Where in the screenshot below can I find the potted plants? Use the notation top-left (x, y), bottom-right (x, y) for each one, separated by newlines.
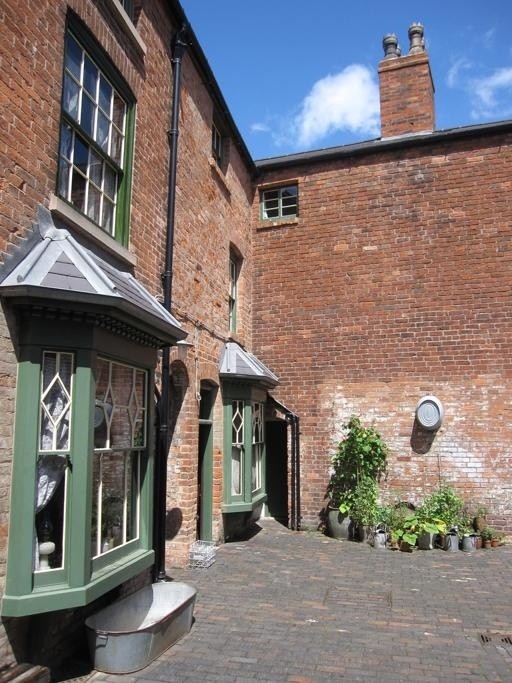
top-left (326, 417), bottom-right (500, 554)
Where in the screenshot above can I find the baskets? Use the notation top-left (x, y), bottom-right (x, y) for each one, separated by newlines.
top-left (189, 540), bottom-right (216, 568)
